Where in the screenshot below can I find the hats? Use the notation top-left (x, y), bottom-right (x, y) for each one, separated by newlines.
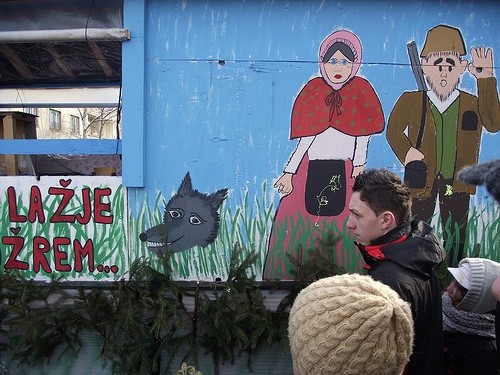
top-left (287, 272), bottom-right (413, 375)
top-left (447, 263), bottom-right (471, 290)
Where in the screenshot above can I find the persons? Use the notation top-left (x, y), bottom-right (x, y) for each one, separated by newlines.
top-left (441, 257), bottom-right (499, 374)
top-left (287, 272), bottom-right (415, 375)
top-left (346, 167), bottom-right (446, 375)
